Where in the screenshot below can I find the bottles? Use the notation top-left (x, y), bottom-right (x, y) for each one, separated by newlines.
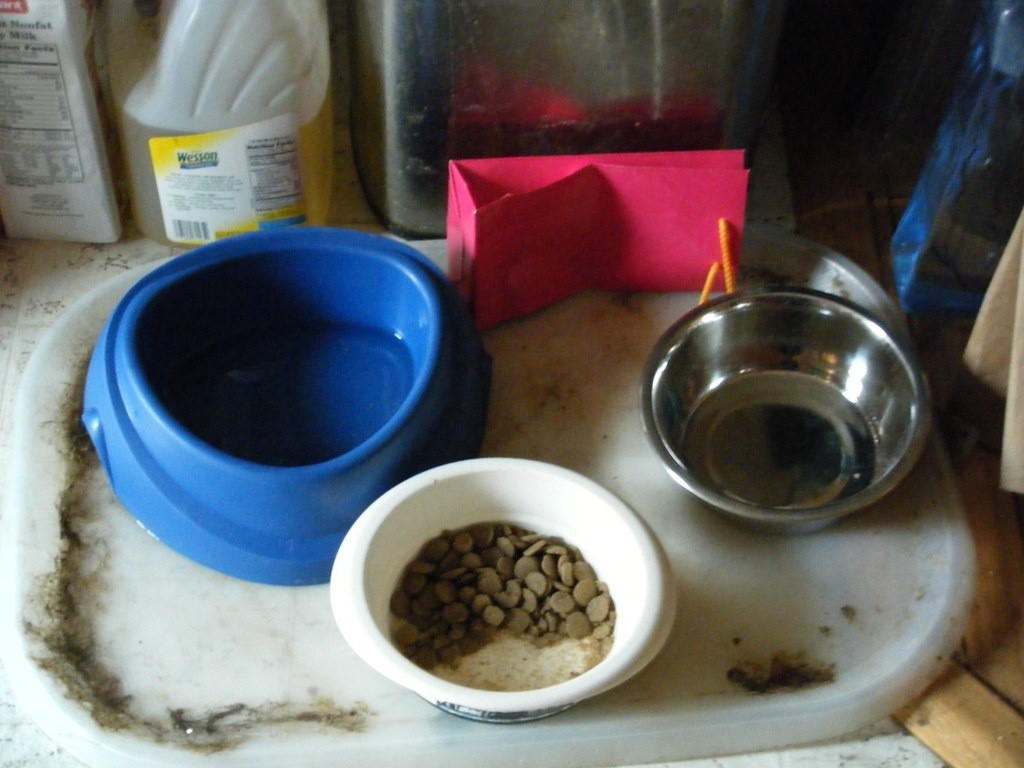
top-left (104, 0), bottom-right (334, 251)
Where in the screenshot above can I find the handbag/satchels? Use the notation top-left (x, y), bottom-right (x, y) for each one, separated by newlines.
top-left (446, 148), bottom-right (749, 335)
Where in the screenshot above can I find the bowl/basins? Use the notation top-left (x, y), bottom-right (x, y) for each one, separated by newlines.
top-left (81, 226), bottom-right (491, 587)
top-left (639, 287), bottom-right (932, 536)
top-left (329, 456), bottom-right (676, 725)
